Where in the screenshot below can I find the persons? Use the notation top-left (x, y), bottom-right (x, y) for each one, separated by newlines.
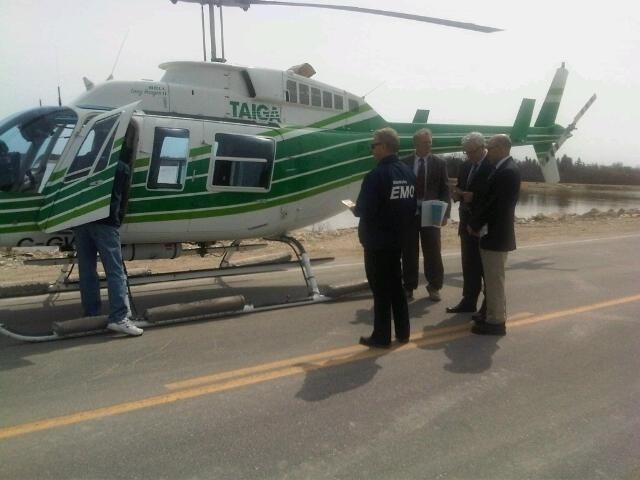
top-left (469, 133), bottom-right (518, 334)
top-left (349, 125), bottom-right (418, 347)
top-left (70, 130), bottom-right (147, 337)
top-left (400, 129), bottom-right (451, 300)
top-left (448, 129), bottom-right (490, 313)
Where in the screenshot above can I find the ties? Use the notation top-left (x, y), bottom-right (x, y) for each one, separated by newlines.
top-left (468, 164), bottom-right (478, 186)
top-left (415, 159), bottom-right (425, 199)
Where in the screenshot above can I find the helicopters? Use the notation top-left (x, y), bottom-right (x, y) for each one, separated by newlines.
top-left (0, 1), bottom-right (599, 342)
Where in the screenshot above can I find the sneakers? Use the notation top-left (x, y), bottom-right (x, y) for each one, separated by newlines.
top-left (107, 318), bottom-right (143, 336)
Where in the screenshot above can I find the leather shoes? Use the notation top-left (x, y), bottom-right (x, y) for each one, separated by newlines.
top-left (359, 285), bottom-right (506, 348)
